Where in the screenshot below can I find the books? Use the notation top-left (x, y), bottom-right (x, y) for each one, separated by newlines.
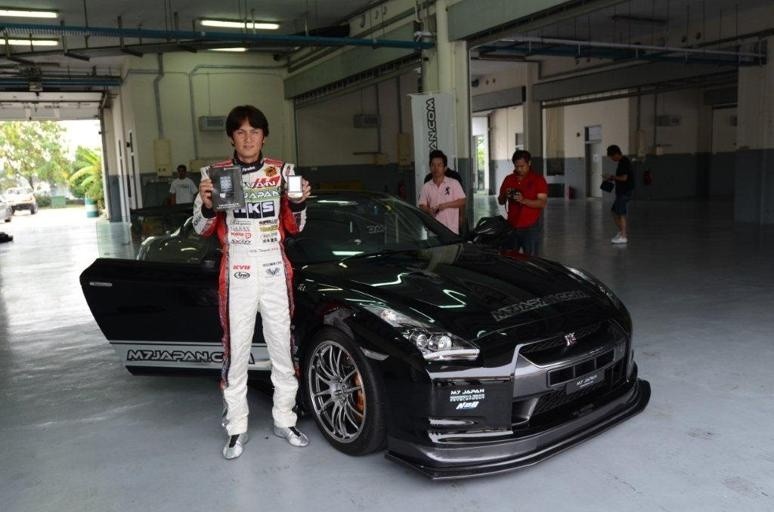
top-left (208, 158), bottom-right (244, 213)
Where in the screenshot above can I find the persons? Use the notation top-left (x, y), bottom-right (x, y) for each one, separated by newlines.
top-left (599, 144), bottom-right (635, 243)
top-left (189, 105), bottom-right (317, 460)
top-left (422, 150), bottom-right (463, 230)
top-left (497, 149), bottom-right (549, 254)
top-left (169, 165), bottom-right (197, 204)
top-left (417, 151), bottom-right (466, 237)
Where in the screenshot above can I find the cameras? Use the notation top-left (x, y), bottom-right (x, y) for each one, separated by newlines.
top-left (507, 188), bottom-right (521, 198)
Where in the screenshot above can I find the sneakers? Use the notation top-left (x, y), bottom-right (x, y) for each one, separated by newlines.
top-left (220, 433), bottom-right (248, 461)
top-left (610, 232), bottom-right (628, 245)
top-left (272, 424), bottom-right (309, 447)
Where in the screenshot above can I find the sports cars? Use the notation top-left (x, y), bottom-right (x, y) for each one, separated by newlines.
top-left (79, 189), bottom-right (651, 480)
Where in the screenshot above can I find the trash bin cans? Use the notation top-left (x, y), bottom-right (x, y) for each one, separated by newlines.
top-left (52, 196), bottom-right (66, 209)
top-left (85, 198), bottom-right (98, 217)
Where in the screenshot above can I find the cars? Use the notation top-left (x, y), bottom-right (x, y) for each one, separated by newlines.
top-left (0, 187), bottom-right (37, 223)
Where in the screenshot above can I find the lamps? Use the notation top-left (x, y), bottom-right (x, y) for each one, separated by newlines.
top-left (0, 37), bottom-right (58, 46)
top-left (198, 16), bottom-right (280, 30)
top-left (0, 6), bottom-right (59, 18)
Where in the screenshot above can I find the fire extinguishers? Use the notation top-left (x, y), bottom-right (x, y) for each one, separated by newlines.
top-left (399, 182), bottom-right (405, 201)
